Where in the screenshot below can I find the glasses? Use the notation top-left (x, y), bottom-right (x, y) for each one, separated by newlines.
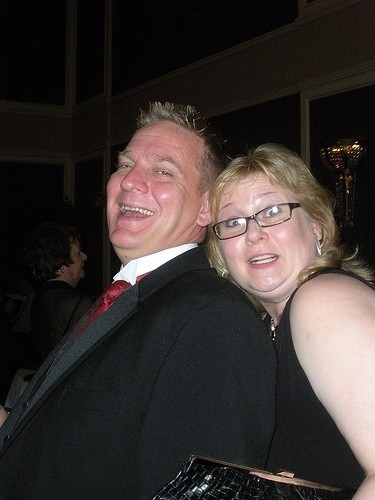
top-left (212, 203), bottom-right (301, 240)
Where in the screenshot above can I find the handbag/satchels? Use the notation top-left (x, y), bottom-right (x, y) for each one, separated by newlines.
top-left (153, 455), bottom-right (357, 500)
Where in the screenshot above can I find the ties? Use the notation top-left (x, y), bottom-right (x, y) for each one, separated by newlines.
top-left (62, 269), bottom-right (153, 351)
